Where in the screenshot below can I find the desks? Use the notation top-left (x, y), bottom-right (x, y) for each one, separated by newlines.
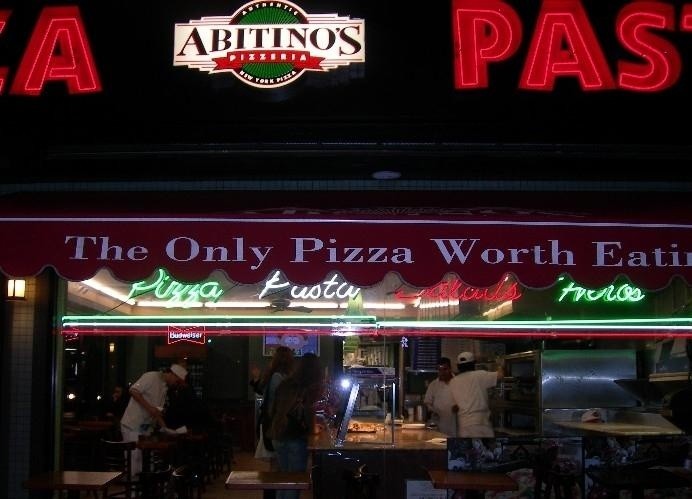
top-left (553, 421), bottom-right (684, 499)
top-left (305, 420), bottom-right (448, 498)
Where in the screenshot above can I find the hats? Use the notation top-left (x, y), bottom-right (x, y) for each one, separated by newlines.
top-left (170, 364), bottom-right (188, 381)
top-left (457, 352), bottom-right (475, 364)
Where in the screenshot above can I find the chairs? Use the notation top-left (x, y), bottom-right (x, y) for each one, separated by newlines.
top-left (99, 420), bottom-right (232, 499)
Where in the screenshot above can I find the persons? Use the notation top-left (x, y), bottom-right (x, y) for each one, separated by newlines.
top-left (424, 351), bottom-right (504, 459)
top-left (120, 364), bottom-right (188, 498)
top-left (262, 346), bottom-right (322, 499)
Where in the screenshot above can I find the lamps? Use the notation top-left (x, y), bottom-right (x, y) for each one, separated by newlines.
top-left (5, 279), bottom-right (26, 299)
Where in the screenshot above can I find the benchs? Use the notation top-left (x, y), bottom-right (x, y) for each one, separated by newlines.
top-left (593, 471), bottom-right (690, 499)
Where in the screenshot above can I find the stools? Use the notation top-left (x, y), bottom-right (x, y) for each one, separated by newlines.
top-left (22, 471), bottom-right (124, 499)
top-left (225, 471), bottom-right (313, 498)
top-left (427, 471), bottom-right (518, 499)
